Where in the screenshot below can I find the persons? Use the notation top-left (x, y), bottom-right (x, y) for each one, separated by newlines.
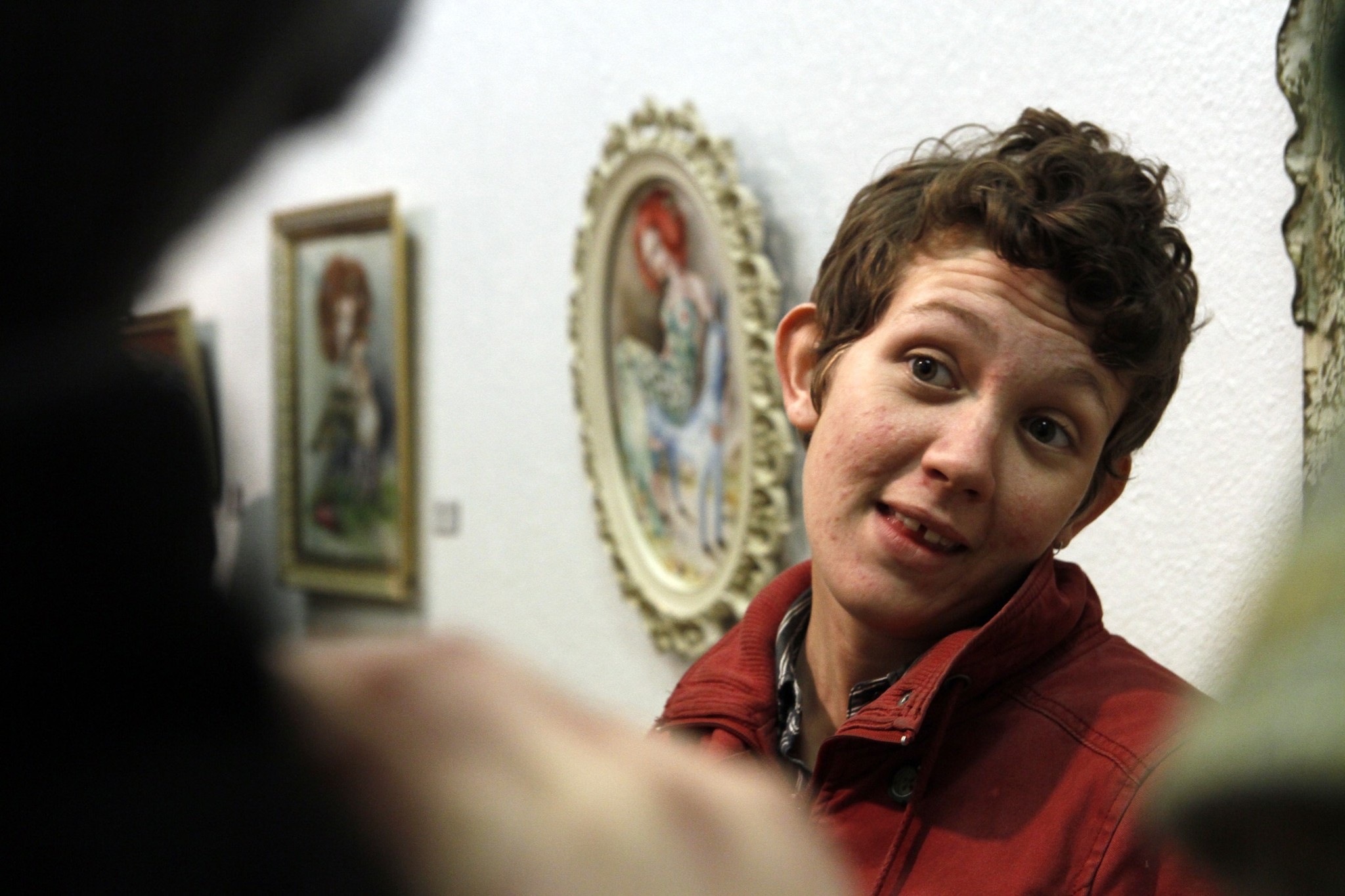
top-left (658, 92), bottom-right (1266, 896)
top-left (0, 1), bottom-right (869, 891)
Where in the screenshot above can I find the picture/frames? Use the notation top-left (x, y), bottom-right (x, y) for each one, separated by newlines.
top-left (119, 307), bottom-right (226, 515)
top-left (564, 90), bottom-right (793, 658)
top-left (266, 190), bottom-right (422, 606)
